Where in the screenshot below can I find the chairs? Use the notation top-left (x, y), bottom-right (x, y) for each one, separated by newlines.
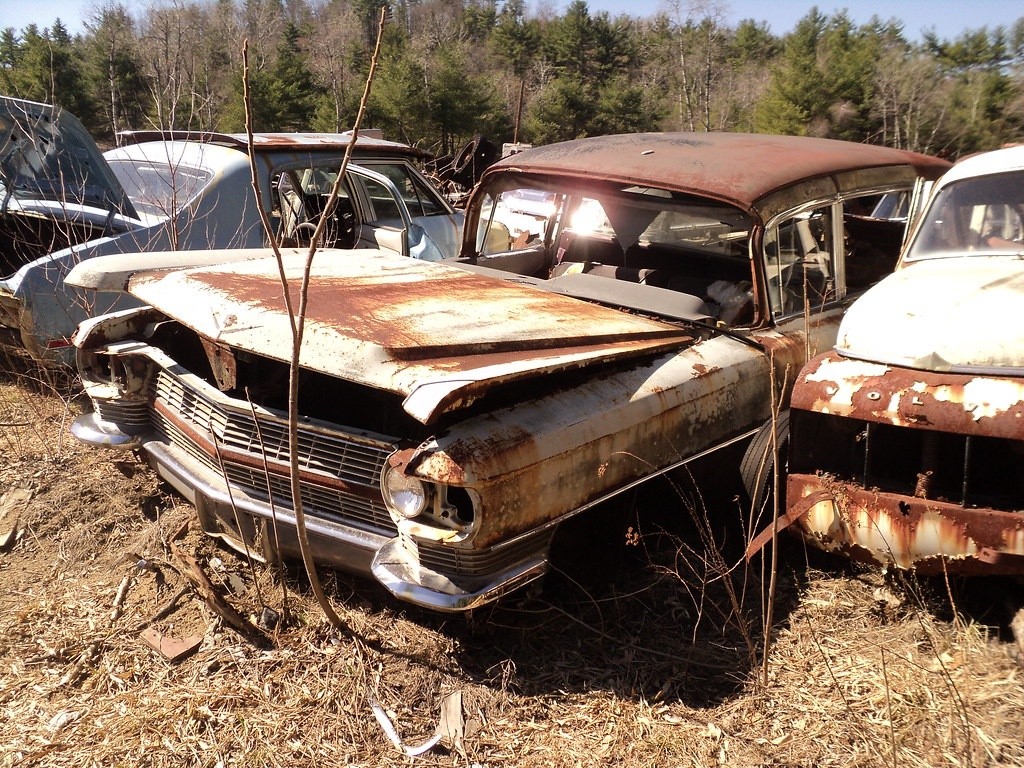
top-left (698, 279), bottom-right (751, 325)
top-left (556, 228), bottom-right (625, 280)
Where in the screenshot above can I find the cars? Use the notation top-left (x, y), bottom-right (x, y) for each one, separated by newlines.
top-left (71, 131), bottom-right (988, 614)
top-left (1, 96), bottom-right (609, 396)
top-left (731, 136), bottom-right (1024, 599)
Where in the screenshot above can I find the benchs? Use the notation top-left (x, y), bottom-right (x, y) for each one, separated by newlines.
top-left (612, 242), bottom-right (750, 289)
